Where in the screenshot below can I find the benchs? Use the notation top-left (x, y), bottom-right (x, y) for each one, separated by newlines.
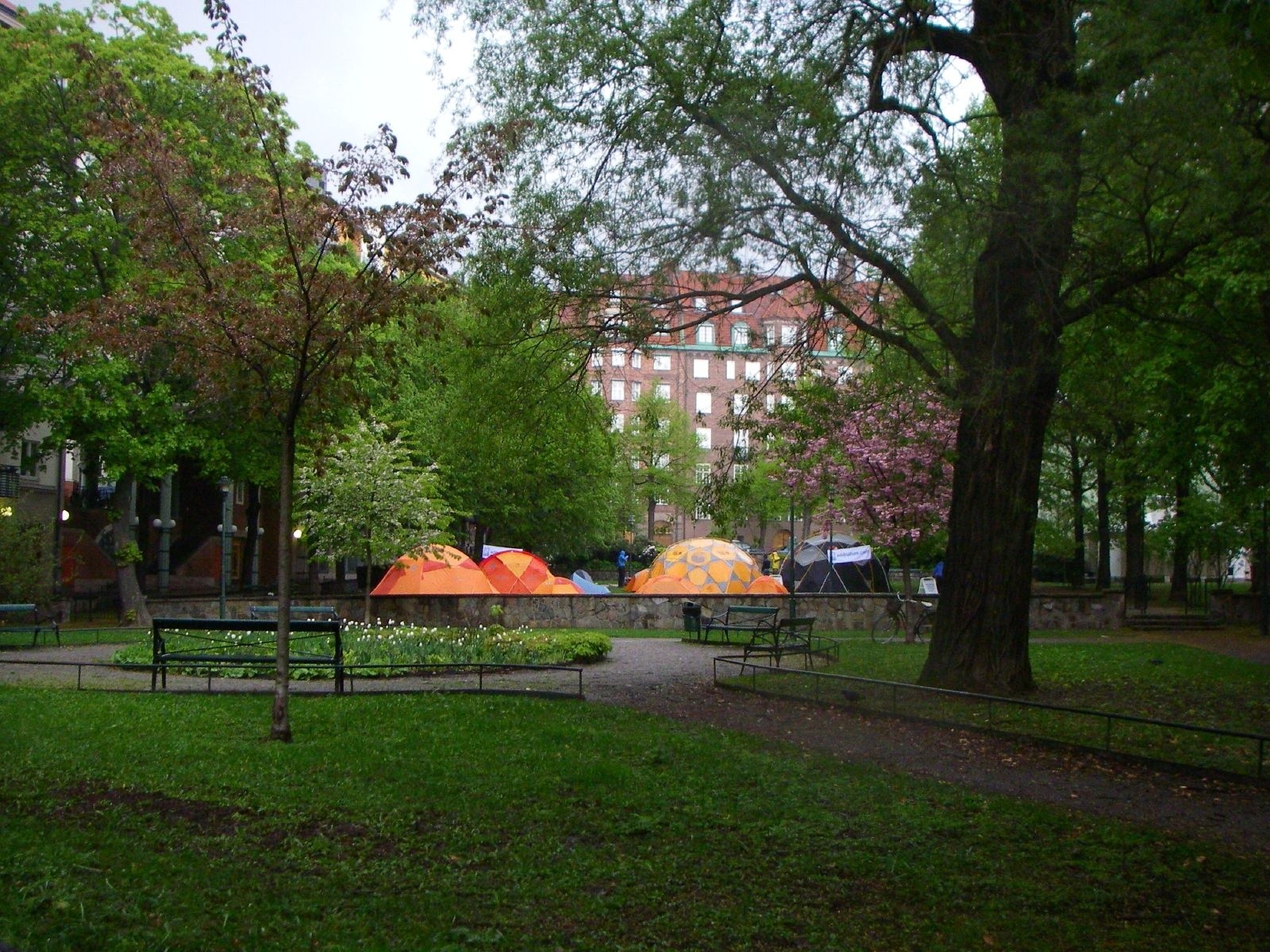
top-left (704, 604), bottom-right (782, 647)
top-left (152, 614), bottom-right (349, 694)
top-left (737, 616), bottom-right (816, 668)
top-left (0, 603), bottom-right (62, 648)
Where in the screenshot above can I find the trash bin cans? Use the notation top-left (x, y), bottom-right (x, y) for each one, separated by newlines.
top-left (682, 601), bottom-right (701, 632)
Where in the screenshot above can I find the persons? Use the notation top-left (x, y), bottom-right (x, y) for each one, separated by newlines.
top-left (750, 550), bottom-right (784, 577)
top-left (932, 558), bottom-right (944, 594)
top-left (617, 549), bottom-right (628, 588)
top-left (882, 553), bottom-right (890, 574)
top-left (644, 542), bottom-right (656, 556)
